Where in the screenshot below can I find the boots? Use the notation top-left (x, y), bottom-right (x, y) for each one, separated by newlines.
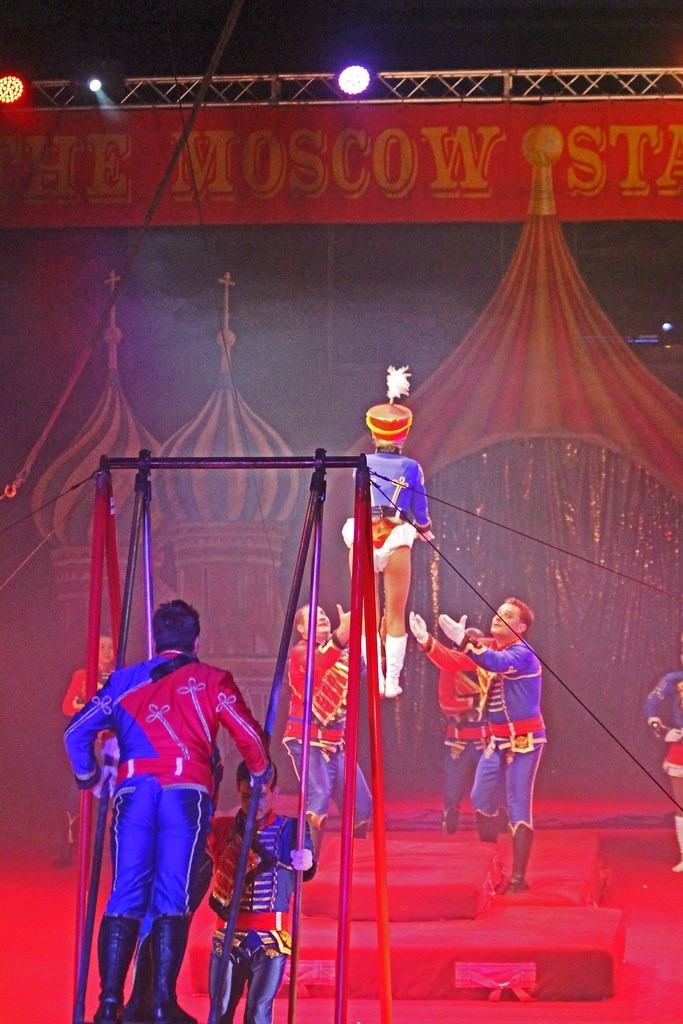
top-left (498, 820), bottom-right (534, 894)
top-left (146, 914), bottom-right (198, 1024)
top-left (306, 812), bottom-right (329, 863)
top-left (51, 811), bottom-right (79, 868)
top-left (672, 816), bottom-right (683, 872)
top-left (475, 810), bottom-right (500, 843)
top-left (353, 820), bottom-right (369, 839)
top-left (122, 932), bottom-right (152, 1024)
top-left (362, 632), bottom-right (385, 694)
top-left (385, 633), bottom-right (409, 698)
top-left (498, 806), bottom-right (509, 833)
top-left (95, 914), bottom-right (139, 1024)
top-left (442, 806), bottom-right (459, 834)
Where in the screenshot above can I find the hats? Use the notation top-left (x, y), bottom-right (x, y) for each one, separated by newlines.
top-left (366, 365), bottom-right (413, 446)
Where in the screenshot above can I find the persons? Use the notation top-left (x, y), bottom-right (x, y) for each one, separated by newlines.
top-left (61, 596), bottom-right (548, 1024)
top-left (642, 652), bottom-right (683, 872)
top-left (340, 404), bottom-right (436, 699)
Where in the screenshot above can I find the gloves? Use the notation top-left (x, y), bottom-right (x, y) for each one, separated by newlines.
top-left (409, 611), bottom-right (430, 645)
top-left (291, 849), bottom-right (313, 870)
top-left (416, 530), bottom-right (435, 543)
top-left (249, 760), bottom-right (274, 794)
top-left (438, 614), bottom-right (468, 646)
top-left (92, 766), bottom-right (117, 799)
